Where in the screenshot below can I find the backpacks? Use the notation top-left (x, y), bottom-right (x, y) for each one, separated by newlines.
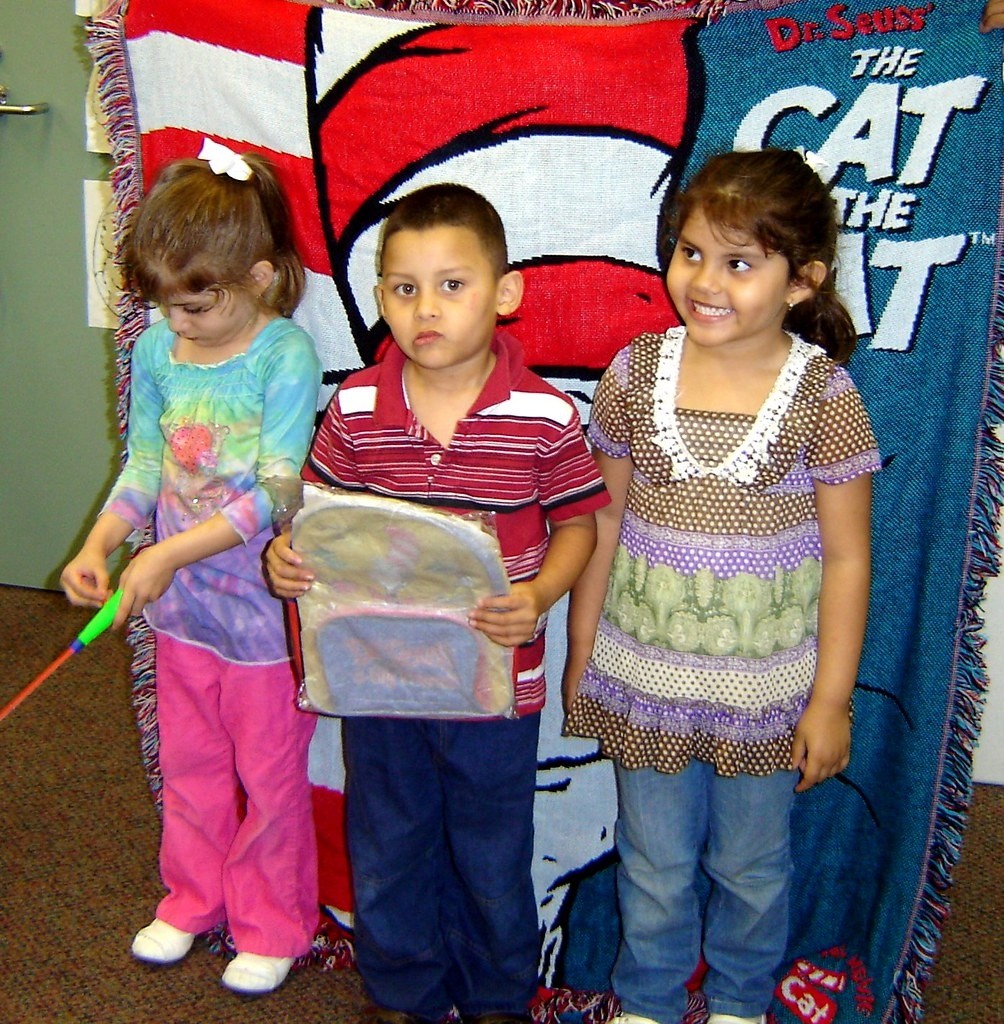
top-left (291, 501), bottom-right (513, 716)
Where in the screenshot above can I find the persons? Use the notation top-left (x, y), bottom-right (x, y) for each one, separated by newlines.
top-left (565, 149), bottom-right (882, 1024)
top-left (61, 137), bottom-right (324, 992)
top-left (268, 184), bottom-right (613, 1024)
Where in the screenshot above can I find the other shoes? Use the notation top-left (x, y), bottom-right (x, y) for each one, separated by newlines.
top-left (132, 917), bottom-right (197, 963)
top-left (223, 951), bottom-right (295, 993)
